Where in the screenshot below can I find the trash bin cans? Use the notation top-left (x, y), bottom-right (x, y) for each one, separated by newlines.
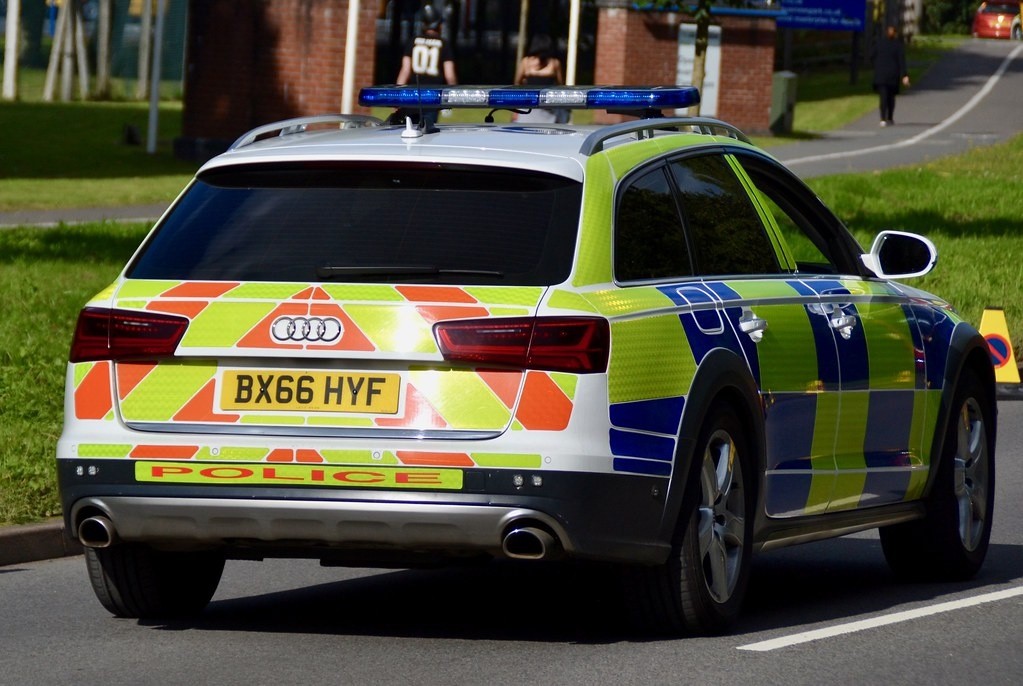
top-left (769, 70), bottom-right (798, 138)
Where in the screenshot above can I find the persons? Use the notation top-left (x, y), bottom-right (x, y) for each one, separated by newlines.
top-left (397, 15), bottom-right (456, 122)
top-left (511, 33), bottom-right (563, 124)
top-left (870, 25), bottom-right (910, 128)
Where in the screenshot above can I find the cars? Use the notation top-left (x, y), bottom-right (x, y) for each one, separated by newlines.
top-left (972, 0), bottom-right (1023, 40)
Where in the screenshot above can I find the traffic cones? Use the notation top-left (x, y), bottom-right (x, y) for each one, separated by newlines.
top-left (978, 305), bottom-right (1023, 401)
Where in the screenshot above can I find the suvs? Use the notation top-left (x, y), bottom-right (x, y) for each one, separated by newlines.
top-left (55, 84), bottom-right (997, 632)
